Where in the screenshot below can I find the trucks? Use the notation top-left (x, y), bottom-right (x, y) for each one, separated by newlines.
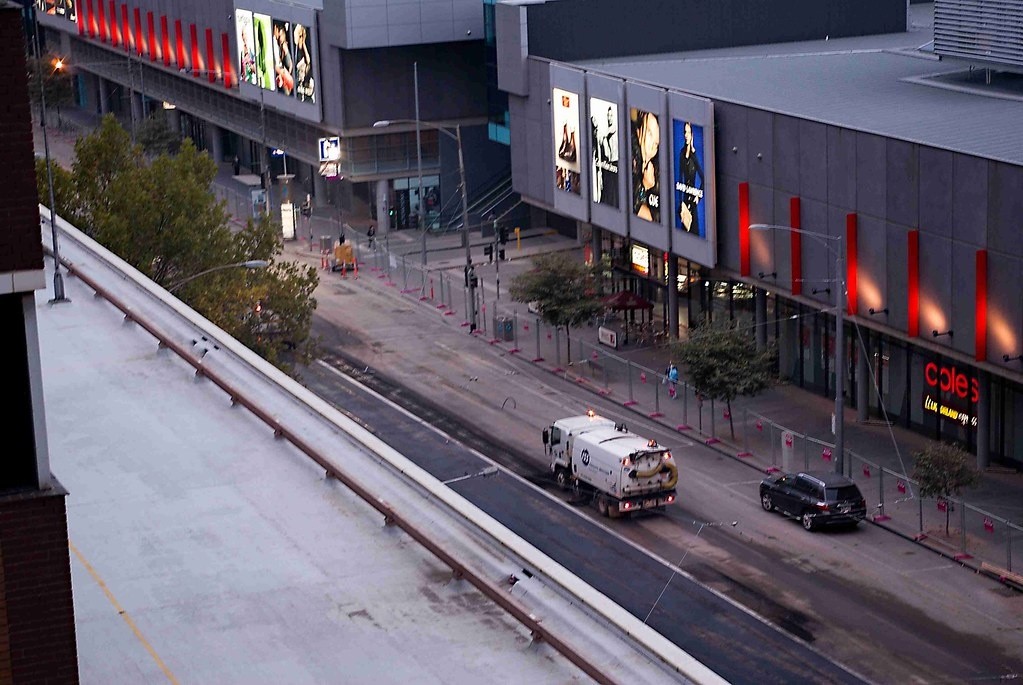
top-left (543, 408), bottom-right (679, 520)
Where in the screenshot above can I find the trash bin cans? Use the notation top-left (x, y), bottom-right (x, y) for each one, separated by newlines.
top-left (497, 315), bottom-right (513, 340)
top-left (425, 211), bottom-right (439, 231)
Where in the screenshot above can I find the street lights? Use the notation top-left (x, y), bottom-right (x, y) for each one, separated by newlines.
top-left (372, 118), bottom-right (474, 270)
top-left (179, 65), bottom-right (273, 219)
top-left (52, 53), bottom-right (148, 124)
top-left (748, 218), bottom-right (849, 475)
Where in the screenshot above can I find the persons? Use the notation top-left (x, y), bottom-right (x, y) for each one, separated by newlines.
top-left (296, 64), bottom-right (315, 102)
top-left (676, 122), bottom-right (704, 236)
top-left (664, 359), bottom-right (680, 400)
top-left (601, 107), bottom-right (619, 208)
top-left (293, 24), bottom-right (311, 74)
top-left (273, 22), bottom-right (284, 93)
top-left (238, 30), bottom-right (255, 85)
top-left (627, 113), bottom-right (660, 223)
top-left (590, 116), bottom-right (607, 203)
top-left (279, 26), bottom-right (293, 95)
top-left (367, 225), bottom-right (377, 252)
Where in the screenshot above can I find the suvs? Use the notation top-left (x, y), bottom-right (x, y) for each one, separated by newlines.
top-left (757, 470), bottom-right (868, 531)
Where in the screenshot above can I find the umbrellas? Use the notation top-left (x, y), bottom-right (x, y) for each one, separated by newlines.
top-left (597, 289), bottom-right (654, 346)
